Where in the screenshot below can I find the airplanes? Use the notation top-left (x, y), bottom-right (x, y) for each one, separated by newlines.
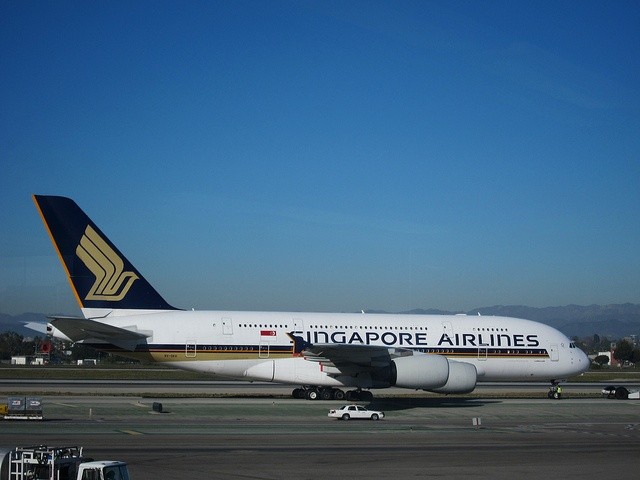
top-left (31, 194), bottom-right (590, 400)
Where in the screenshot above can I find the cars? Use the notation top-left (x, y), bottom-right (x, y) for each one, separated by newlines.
top-left (328, 404), bottom-right (384, 421)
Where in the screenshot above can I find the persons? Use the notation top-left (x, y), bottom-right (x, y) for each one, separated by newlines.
top-left (556, 385), bottom-right (562, 400)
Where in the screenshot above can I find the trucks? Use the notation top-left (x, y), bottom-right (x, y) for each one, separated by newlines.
top-left (0, 444), bottom-right (130, 480)
top-left (0, 396), bottom-right (43, 421)
